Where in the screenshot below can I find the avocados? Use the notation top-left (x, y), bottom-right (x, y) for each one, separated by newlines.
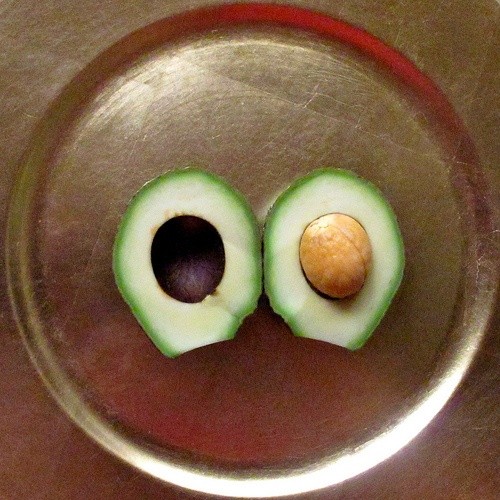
top-left (113, 164), bottom-right (404, 360)
